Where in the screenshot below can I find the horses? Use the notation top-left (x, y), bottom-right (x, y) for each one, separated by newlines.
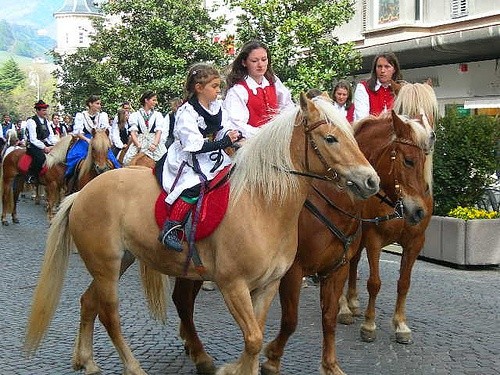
top-left (22, 92), bottom-right (381, 375)
top-left (0, 124), bottom-right (158, 224)
top-left (335, 78), bottom-right (440, 345)
top-left (171, 109), bottom-right (436, 375)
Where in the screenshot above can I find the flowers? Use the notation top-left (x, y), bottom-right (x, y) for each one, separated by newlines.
top-left (446, 205), bottom-right (500, 221)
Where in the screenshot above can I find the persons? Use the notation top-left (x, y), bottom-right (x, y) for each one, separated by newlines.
top-left (64, 95), bottom-right (123, 188)
top-left (61, 114), bottom-right (74, 134)
top-left (331, 81), bottom-right (355, 125)
top-left (50, 113), bottom-right (68, 143)
top-left (112, 109), bottom-right (131, 159)
top-left (353, 53), bottom-right (403, 123)
top-left (109, 117), bottom-right (114, 126)
top-left (158, 62), bottom-right (246, 253)
top-left (0, 115), bottom-right (31, 145)
top-left (122, 91), bottom-right (167, 167)
top-left (112, 102), bottom-right (131, 125)
top-left (165, 96), bottom-right (184, 147)
top-left (223, 41), bottom-right (296, 150)
top-left (24, 99), bottom-right (55, 187)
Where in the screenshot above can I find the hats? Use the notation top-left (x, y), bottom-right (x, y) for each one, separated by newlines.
top-left (34, 100), bottom-right (49, 109)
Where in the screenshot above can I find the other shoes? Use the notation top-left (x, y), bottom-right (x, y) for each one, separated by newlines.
top-left (64, 176), bottom-right (74, 195)
top-left (158, 218), bottom-right (185, 252)
top-left (28, 172), bottom-right (39, 184)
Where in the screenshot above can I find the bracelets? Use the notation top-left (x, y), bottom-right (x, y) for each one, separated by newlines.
top-left (152, 143), bottom-right (157, 147)
